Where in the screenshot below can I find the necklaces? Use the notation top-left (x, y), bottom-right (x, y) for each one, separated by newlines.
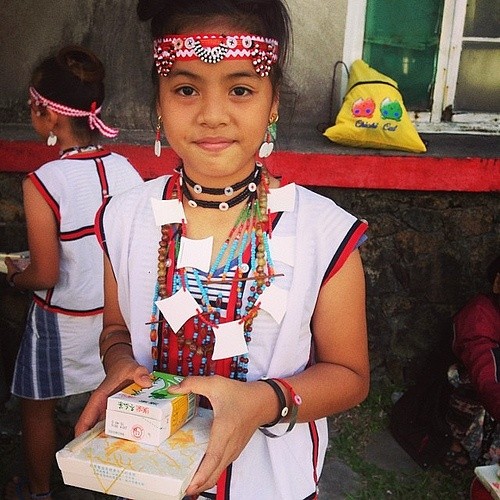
top-left (149, 159), bottom-right (275, 411)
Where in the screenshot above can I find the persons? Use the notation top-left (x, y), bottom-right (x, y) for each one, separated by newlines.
top-left (0, 46), bottom-right (144, 500)
top-left (428, 257), bottom-right (500, 500)
top-left (74, 0), bottom-right (369, 499)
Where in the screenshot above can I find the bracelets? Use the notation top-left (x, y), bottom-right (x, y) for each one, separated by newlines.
top-left (99, 327), bottom-right (132, 364)
top-left (257, 378), bottom-right (301, 438)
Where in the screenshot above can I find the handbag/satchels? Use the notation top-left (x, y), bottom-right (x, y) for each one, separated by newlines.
top-left (323, 57), bottom-right (427, 153)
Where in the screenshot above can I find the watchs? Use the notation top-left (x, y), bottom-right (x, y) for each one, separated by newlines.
top-left (9, 271), bottom-right (22, 287)
